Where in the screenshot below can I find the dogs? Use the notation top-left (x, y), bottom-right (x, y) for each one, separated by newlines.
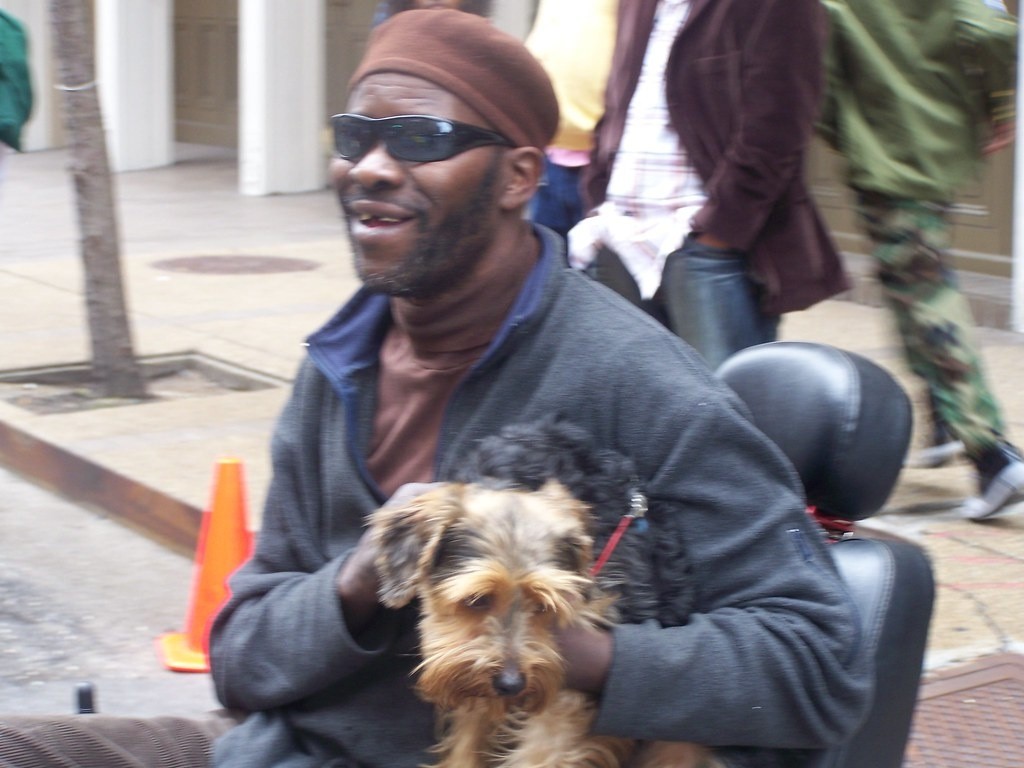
top-left (361, 415), bottom-right (733, 768)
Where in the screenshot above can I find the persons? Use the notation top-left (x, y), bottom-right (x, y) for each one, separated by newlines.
top-left (201, 11), bottom-right (876, 768)
top-left (523, 2), bottom-right (1024, 525)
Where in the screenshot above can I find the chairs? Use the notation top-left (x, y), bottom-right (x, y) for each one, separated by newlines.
top-left (713, 343), bottom-right (938, 768)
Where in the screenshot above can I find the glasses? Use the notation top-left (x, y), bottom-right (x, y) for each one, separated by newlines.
top-left (330, 109), bottom-right (517, 166)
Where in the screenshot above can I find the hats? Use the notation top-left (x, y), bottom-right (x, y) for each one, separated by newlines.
top-left (347, 8), bottom-right (560, 151)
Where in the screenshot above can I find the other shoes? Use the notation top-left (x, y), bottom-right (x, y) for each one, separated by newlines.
top-left (955, 462), bottom-right (1024, 519)
top-left (901, 431), bottom-right (971, 469)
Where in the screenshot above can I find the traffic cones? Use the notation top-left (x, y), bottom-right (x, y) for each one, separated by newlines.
top-left (160, 457), bottom-right (256, 672)
